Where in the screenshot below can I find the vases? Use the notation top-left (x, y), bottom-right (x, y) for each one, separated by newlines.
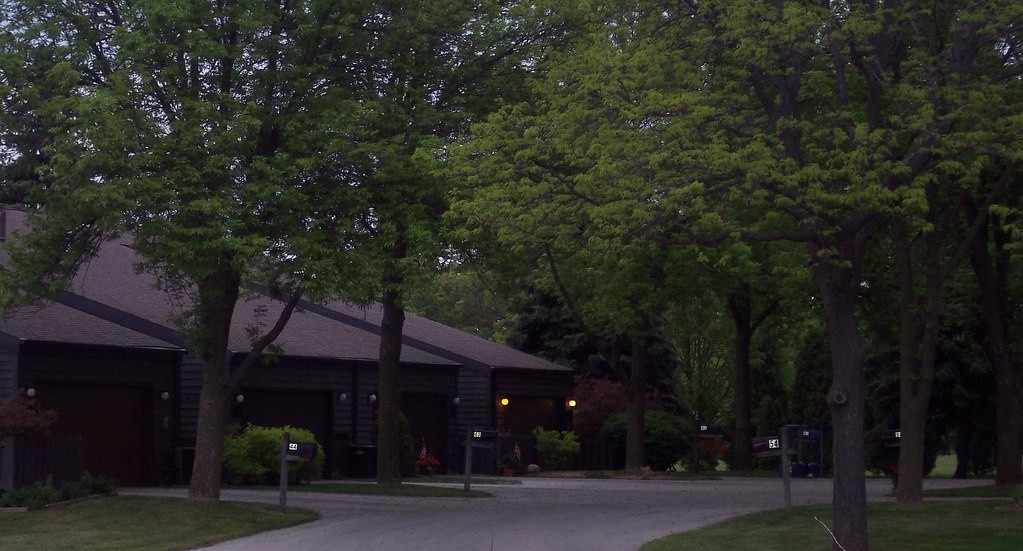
top-left (502, 465), bottom-right (517, 476)
top-left (420, 465), bottom-right (435, 475)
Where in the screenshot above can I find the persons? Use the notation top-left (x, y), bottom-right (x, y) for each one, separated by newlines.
top-left (885, 463), bottom-right (898, 488)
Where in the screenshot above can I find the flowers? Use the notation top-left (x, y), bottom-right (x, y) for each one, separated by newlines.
top-left (414, 456), bottom-right (440, 467)
top-left (495, 450), bottom-right (513, 465)
